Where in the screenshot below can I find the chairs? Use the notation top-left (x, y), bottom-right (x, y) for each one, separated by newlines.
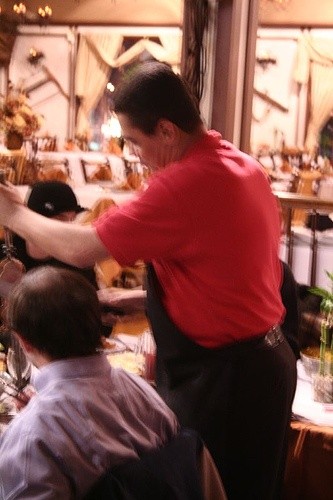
top-left (72, 426), bottom-right (206, 500)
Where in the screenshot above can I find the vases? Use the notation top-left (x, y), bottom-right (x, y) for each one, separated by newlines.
top-left (3, 131), bottom-right (22, 149)
top-left (300, 347), bottom-right (333, 402)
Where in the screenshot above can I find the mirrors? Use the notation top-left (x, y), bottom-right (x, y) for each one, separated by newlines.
top-left (0, 0), bottom-right (333, 208)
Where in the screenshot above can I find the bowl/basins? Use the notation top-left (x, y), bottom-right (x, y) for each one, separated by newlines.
top-left (299, 350), bottom-right (333, 376)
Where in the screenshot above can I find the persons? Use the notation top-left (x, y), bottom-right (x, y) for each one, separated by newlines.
top-left (0, 181), bottom-right (100, 291)
top-left (145, 257), bottom-right (301, 401)
top-left (0, 63), bottom-right (297, 500)
top-left (1, 264), bottom-right (181, 500)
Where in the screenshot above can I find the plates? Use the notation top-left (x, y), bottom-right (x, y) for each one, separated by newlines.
top-left (105, 352), bottom-right (146, 375)
top-left (96, 338), bottom-right (125, 353)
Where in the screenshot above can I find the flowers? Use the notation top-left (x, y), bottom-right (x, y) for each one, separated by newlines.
top-left (1, 90), bottom-right (41, 138)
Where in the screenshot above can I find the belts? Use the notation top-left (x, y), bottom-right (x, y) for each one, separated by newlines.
top-left (202, 322), bottom-right (287, 358)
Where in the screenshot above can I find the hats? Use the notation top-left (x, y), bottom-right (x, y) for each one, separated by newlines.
top-left (28, 180), bottom-right (87, 219)
